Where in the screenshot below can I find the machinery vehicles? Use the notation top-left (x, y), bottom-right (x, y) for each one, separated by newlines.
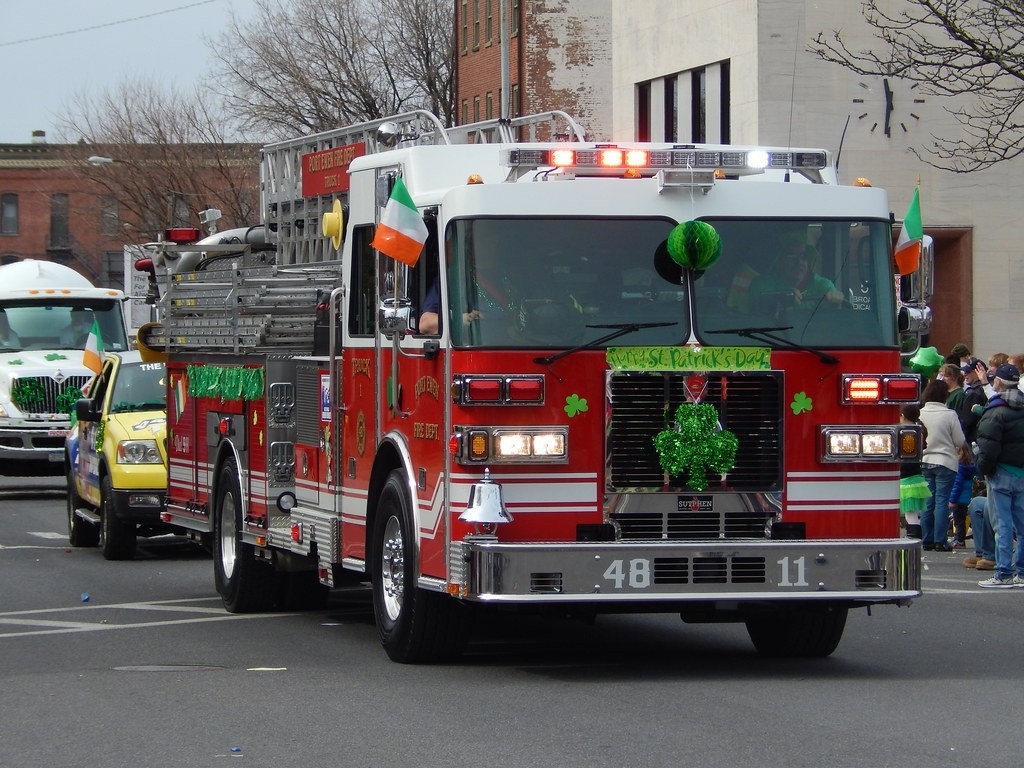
top-left (0, 258), bottom-right (129, 478)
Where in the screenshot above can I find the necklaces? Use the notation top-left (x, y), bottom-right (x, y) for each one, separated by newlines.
top-left (471, 267), bottom-right (517, 314)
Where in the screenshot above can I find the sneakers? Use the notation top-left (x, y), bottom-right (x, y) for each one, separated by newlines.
top-left (978, 577), bottom-right (1013, 588)
top-left (951, 540), bottom-right (966, 549)
top-left (924, 542), bottom-right (951, 552)
top-left (963, 557), bottom-right (995, 570)
top-left (1013, 575), bottom-right (1024, 588)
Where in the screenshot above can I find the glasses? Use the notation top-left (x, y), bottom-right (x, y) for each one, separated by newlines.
top-left (788, 252), bottom-right (806, 260)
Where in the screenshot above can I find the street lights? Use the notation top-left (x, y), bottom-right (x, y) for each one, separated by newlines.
top-left (89, 155), bottom-right (166, 245)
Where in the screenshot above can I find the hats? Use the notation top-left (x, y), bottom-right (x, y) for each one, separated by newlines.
top-left (951, 344), bottom-right (971, 357)
top-left (957, 359), bottom-right (988, 372)
top-left (994, 364), bottom-right (1020, 382)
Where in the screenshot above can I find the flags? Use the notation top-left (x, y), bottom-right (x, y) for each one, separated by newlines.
top-left (894, 187), bottom-right (923, 277)
top-left (83, 318), bottom-right (106, 376)
top-left (368, 172), bottom-right (429, 268)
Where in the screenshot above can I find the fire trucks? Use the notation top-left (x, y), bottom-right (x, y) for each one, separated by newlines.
top-left (131, 109), bottom-right (948, 659)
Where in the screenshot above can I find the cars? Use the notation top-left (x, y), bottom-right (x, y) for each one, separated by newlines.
top-left (65, 348), bottom-right (171, 560)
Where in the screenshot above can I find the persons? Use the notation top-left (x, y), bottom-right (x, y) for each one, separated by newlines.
top-left (0, 307), bottom-right (21, 350)
top-left (899, 341), bottom-right (1024, 589)
top-left (60, 307), bottom-right (92, 345)
top-left (750, 237), bottom-right (837, 318)
top-left (418, 220), bottom-right (583, 335)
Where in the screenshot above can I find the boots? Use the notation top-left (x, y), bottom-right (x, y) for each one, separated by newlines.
top-left (906, 524), bottom-right (923, 541)
